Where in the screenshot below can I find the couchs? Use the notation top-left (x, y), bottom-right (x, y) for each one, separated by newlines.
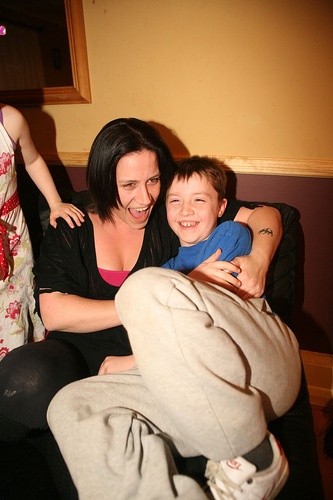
top-left (19, 168), bottom-right (333, 500)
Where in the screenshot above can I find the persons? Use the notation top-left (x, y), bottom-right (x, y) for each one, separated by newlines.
top-left (45, 155), bottom-right (303, 500)
top-left (0, 116), bottom-right (282, 500)
top-left (0, 104), bottom-right (86, 363)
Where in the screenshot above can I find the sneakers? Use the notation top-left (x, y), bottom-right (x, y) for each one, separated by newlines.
top-left (204, 428), bottom-right (290, 500)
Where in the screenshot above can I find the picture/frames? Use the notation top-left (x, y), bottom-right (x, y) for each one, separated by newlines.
top-left (0, 2), bottom-right (93, 106)
